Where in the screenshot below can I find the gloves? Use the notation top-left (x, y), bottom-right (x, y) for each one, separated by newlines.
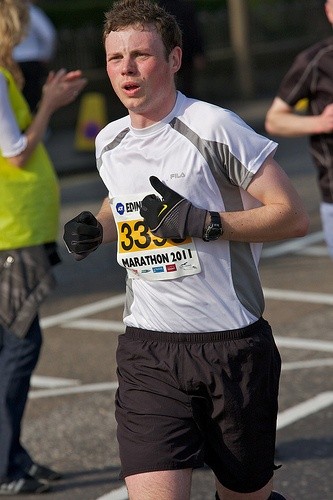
top-left (62, 211), bottom-right (104, 260)
top-left (138, 176), bottom-right (206, 241)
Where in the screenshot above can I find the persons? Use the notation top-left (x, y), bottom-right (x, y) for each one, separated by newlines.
top-left (0, 0), bottom-right (88, 496)
top-left (264, 0), bottom-right (333, 260)
top-left (62, 0), bottom-right (309, 500)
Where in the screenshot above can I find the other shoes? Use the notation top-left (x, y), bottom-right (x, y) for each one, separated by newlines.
top-left (0, 474), bottom-right (50, 496)
top-left (28, 462), bottom-right (62, 482)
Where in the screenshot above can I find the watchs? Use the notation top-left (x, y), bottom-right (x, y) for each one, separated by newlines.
top-left (203, 210), bottom-right (224, 243)
